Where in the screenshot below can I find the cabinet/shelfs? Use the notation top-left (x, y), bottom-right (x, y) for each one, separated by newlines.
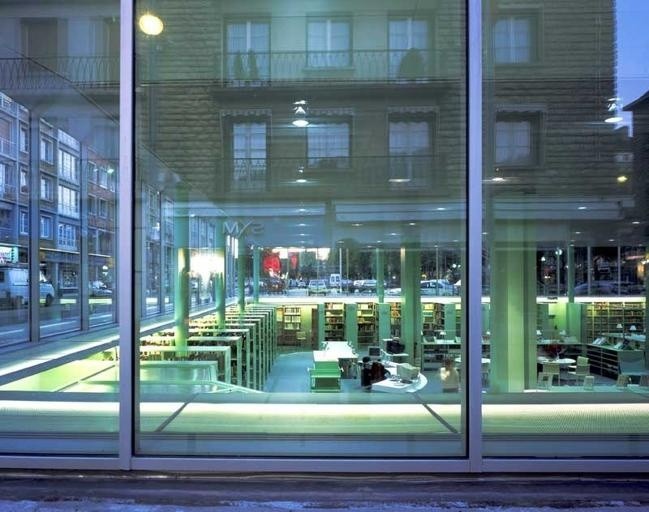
top-left (87, 303), bottom-right (277, 391)
top-left (537, 301), bottom-right (649, 383)
top-left (421, 339), bottom-right (491, 371)
top-left (275, 302), bottom-right (461, 350)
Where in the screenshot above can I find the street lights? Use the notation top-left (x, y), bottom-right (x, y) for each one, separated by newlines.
top-left (540, 257), bottom-right (546, 276)
top-left (553, 247), bottom-right (563, 297)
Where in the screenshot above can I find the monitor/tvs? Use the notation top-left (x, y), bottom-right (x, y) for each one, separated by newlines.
top-left (397, 363), bottom-right (419, 383)
top-left (367, 346), bottom-right (383, 360)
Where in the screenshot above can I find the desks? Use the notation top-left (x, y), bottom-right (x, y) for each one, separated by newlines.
top-left (380, 348), bottom-right (409, 363)
top-left (322, 340), bottom-right (357, 377)
top-left (307, 350), bottom-right (342, 393)
top-left (454, 357), bottom-right (491, 387)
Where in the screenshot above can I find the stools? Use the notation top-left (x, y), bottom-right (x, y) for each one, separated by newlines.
top-left (481, 370), bottom-right (489, 386)
top-left (583, 376), bottom-right (595, 390)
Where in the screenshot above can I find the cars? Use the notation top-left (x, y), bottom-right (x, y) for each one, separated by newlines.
top-left (190, 270), bottom-right (457, 298)
top-left (563, 253), bottom-right (646, 296)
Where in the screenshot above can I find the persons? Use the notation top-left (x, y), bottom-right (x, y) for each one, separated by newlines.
top-left (264, 279), bottom-right (289, 296)
top-left (363, 357), bottom-right (391, 393)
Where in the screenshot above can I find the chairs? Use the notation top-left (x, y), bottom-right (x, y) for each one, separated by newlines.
top-left (538, 363), bottom-right (560, 390)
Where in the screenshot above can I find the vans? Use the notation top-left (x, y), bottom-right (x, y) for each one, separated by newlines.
top-left (0, 261), bottom-right (55, 307)
